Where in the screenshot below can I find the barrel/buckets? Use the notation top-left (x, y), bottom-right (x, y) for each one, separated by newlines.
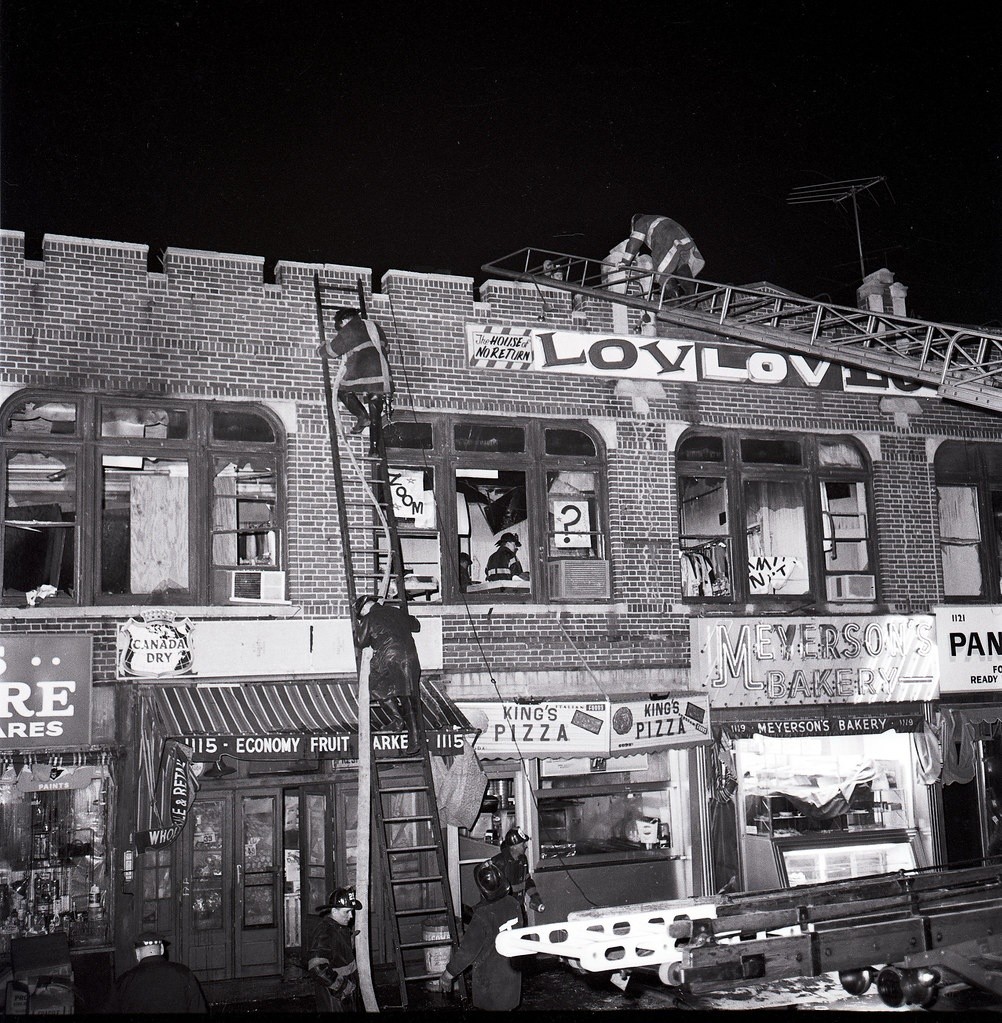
top-left (423, 915), bottom-right (460, 992)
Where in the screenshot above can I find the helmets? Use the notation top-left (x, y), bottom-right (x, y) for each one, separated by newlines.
top-left (473, 860), bottom-right (509, 901)
top-left (504, 827), bottom-right (530, 846)
top-left (314, 888), bottom-right (362, 910)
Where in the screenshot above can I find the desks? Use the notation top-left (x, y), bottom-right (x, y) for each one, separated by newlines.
top-left (465, 580), bottom-right (530, 594)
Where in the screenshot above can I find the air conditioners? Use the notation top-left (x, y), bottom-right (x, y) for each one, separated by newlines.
top-left (825, 574), bottom-right (877, 602)
top-left (545, 559), bottom-right (612, 602)
top-left (229, 569), bottom-right (288, 603)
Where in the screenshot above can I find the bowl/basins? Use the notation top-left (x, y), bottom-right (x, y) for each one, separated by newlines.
top-left (779, 812), bottom-right (792, 816)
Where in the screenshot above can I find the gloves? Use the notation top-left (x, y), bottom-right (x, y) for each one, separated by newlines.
top-left (530, 894), bottom-right (542, 905)
top-left (346, 987), bottom-right (359, 1002)
top-left (438, 975), bottom-right (452, 992)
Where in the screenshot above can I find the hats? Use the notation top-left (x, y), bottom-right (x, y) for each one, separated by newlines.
top-left (134, 932), bottom-right (163, 948)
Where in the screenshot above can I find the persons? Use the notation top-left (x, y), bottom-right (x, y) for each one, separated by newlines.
top-left (354, 595), bottom-right (421, 755)
top-left (438, 827), bottom-right (544, 1012)
top-left (459, 552), bottom-right (482, 592)
top-left (99, 931), bottom-right (208, 1013)
top-left (616, 213), bottom-right (706, 311)
top-left (543, 260), bottom-right (563, 281)
top-left (307, 887), bottom-right (362, 1012)
top-left (485, 532), bottom-right (530, 582)
top-left (316, 307), bottom-right (394, 458)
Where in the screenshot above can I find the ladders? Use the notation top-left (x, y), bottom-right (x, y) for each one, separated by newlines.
top-left (311, 269), bottom-right (467, 1008)
